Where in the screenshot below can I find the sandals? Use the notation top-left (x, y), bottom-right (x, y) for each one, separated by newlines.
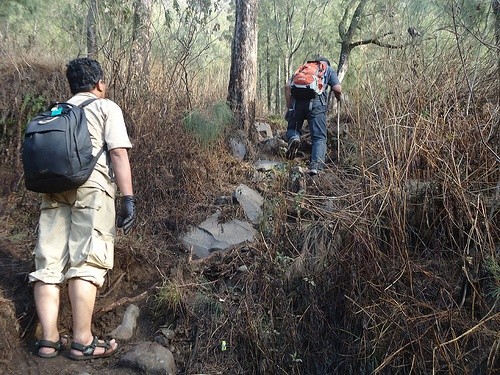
top-left (68, 335), bottom-right (119, 359)
top-left (34, 334), bottom-right (71, 358)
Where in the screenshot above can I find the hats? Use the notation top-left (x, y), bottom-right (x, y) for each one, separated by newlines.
top-left (315, 57), bottom-right (330, 66)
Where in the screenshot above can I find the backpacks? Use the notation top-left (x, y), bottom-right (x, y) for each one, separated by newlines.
top-left (22, 98), bottom-right (109, 193)
top-left (289, 60), bottom-right (329, 99)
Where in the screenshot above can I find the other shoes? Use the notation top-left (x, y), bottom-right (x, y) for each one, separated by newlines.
top-left (285, 138), bottom-right (300, 160)
top-left (309, 168), bottom-right (325, 176)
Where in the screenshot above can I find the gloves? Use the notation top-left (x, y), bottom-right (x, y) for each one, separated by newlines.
top-left (285, 106), bottom-right (294, 122)
top-left (117, 195), bottom-right (136, 235)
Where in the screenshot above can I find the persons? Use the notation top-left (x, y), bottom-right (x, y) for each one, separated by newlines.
top-left (29, 60), bottom-right (136, 360)
top-left (283, 57), bottom-right (343, 174)
